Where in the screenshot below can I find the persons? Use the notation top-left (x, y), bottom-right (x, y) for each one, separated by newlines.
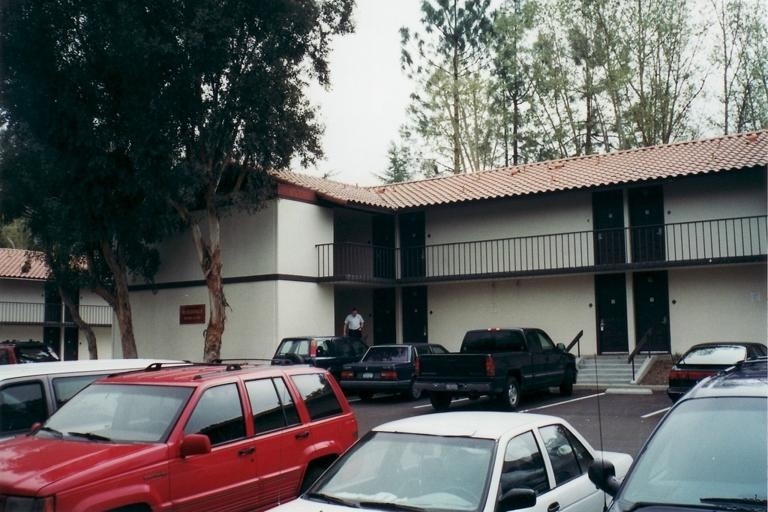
top-left (343, 308), bottom-right (365, 341)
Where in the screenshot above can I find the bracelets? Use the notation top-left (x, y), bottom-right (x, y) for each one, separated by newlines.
top-left (360, 327), bottom-right (362, 329)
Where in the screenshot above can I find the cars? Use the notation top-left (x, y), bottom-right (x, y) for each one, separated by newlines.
top-left (603, 356), bottom-right (767, 512)
top-left (265, 408), bottom-right (632, 512)
top-left (416, 327), bottom-right (577, 410)
top-left (669, 340), bottom-right (767, 403)
top-left (270, 336), bottom-right (449, 400)
top-left (0, 340), bottom-right (60, 363)
